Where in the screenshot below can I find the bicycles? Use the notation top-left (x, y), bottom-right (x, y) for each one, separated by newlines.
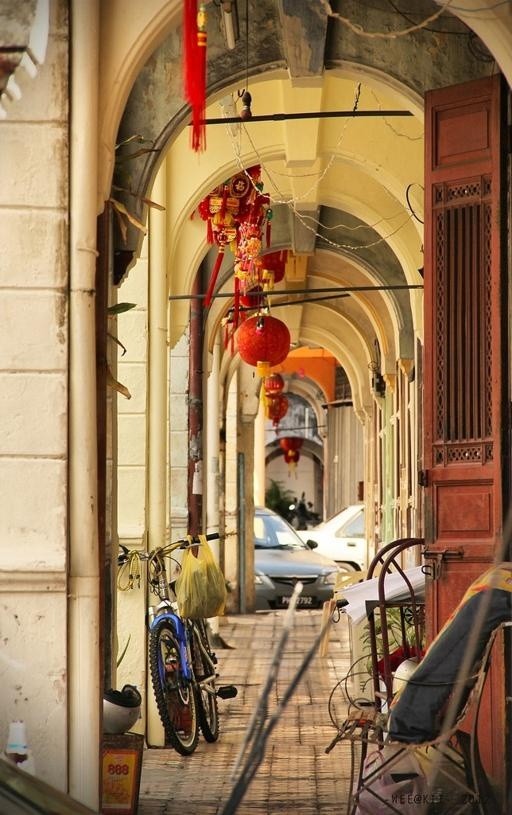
top-left (118, 532), bottom-right (238, 758)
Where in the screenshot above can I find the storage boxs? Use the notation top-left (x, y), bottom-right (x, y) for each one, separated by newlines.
top-left (101, 733), bottom-right (145, 814)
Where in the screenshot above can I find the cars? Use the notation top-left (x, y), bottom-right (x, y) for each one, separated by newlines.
top-left (251, 505), bottom-right (343, 608)
top-left (268, 504), bottom-right (367, 590)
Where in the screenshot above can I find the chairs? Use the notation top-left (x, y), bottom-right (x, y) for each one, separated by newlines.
top-left (324, 559), bottom-right (512, 814)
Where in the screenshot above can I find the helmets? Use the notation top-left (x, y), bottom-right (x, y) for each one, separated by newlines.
top-left (101, 686), bottom-right (142, 735)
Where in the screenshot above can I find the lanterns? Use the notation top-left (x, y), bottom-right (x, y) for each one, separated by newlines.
top-left (280, 436), bottom-right (305, 457)
top-left (282, 451), bottom-right (301, 479)
top-left (195, 161), bottom-right (290, 428)
